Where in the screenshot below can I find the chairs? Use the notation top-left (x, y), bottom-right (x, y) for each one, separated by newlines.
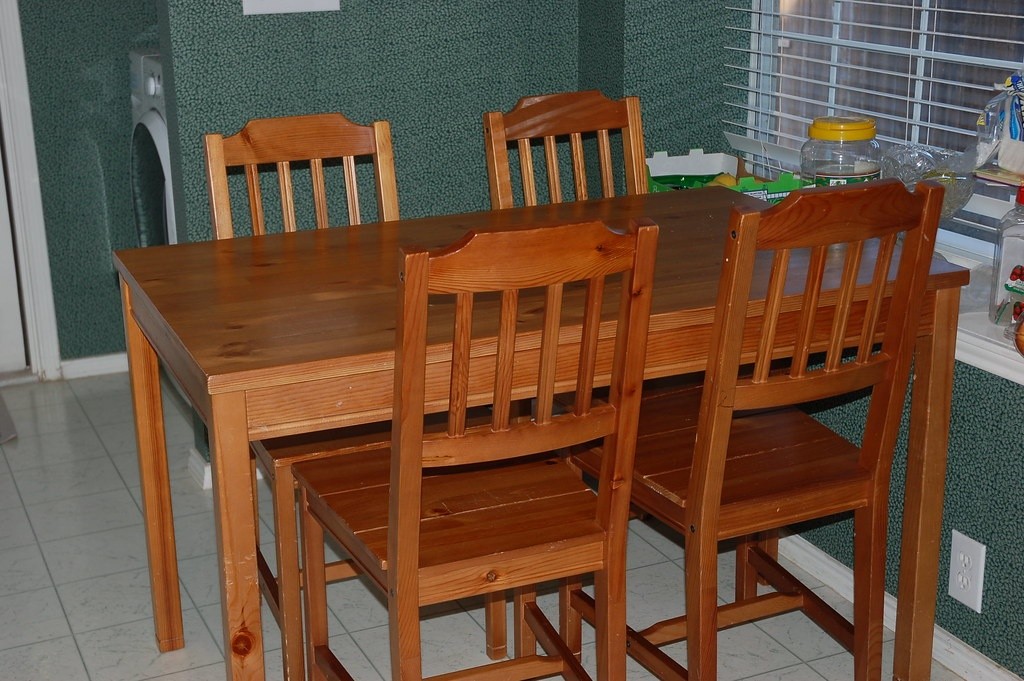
top-left (204, 87), bottom-right (943, 681)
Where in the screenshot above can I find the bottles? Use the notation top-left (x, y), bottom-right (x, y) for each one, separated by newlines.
top-left (987, 183), bottom-right (1024, 326)
top-left (799, 117), bottom-right (881, 189)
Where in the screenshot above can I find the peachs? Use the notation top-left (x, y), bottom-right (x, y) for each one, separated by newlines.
top-left (713, 172), bottom-right (736, 186)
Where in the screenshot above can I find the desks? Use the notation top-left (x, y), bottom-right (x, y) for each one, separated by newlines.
top-left (111, 186), bottom-right (972, 681)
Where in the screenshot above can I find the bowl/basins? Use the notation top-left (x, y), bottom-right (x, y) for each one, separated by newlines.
top-left (882, 144), bottom-right (978, 226)
